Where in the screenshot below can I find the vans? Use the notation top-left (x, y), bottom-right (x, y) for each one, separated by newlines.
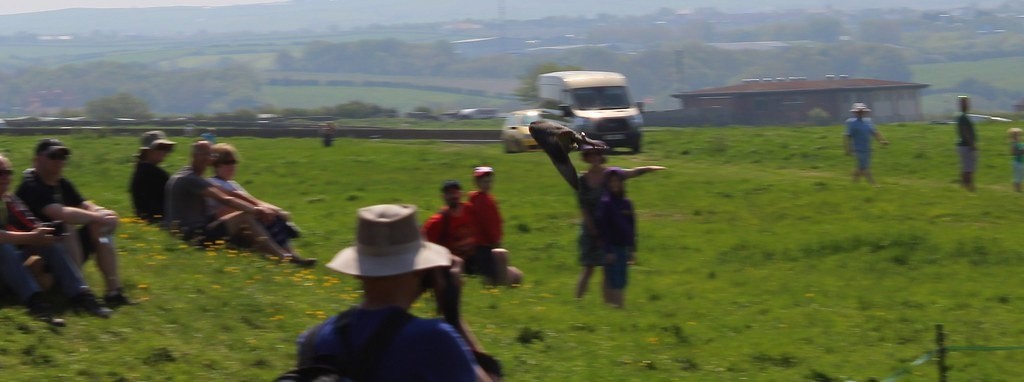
top-left (537, 70), bottom-right (643, 153)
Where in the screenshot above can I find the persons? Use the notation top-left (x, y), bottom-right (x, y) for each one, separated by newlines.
top-left (201, 128), bottom-right (215, 146)
top-left (419, 180), bottom-right (525, 287)
top-left (0, 155), bottom-right (115, 326)
top-left (204, 142), bottom-right (318, 266)
top-left (467, 166), bottom-right (503, 250)
top-left (575, 148), bottom-right (668, 301)
top-left (594, 167), bottom-right (635, 310)
top-left (163, 139), bottom-right (292, 262)
top-left (1008, 128), bottom-right (1024, 192)
top-left (14, 137), bottom-right (148, 317)
top-left (130, 130), bottom-right (178, 224)
top-left (955, 96), bottom-right (978, 194)
top-left (296, 204), bottom-right (504, 382)
top-left (842, 102), bottom-right (891, 185)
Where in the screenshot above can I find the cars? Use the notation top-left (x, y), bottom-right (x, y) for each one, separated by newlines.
top-left (502, 109), bottom-right (546, 154)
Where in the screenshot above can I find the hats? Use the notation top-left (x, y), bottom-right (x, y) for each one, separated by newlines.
top-left (323, 203), bottom-right (452, 277)
top-left (138, 130), bottom-right (177, 156)
top-left (33, 139), bottom-right (71, 157)
top-left (474, 166), bottom-right (494, 176)
top-left (849, 103), bottom-right (872, 112)
top-left (441, 180), bottom-right (461, 191)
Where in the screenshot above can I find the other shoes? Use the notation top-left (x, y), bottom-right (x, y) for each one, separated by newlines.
top-left (72, 294), bottom-right (115, 317)
top-left (34, 313), bottom-right (68, 327)
top-left (284, 250), bottom-right (319, 267)
top-left (102, 287), bottom-right (140, 307)
